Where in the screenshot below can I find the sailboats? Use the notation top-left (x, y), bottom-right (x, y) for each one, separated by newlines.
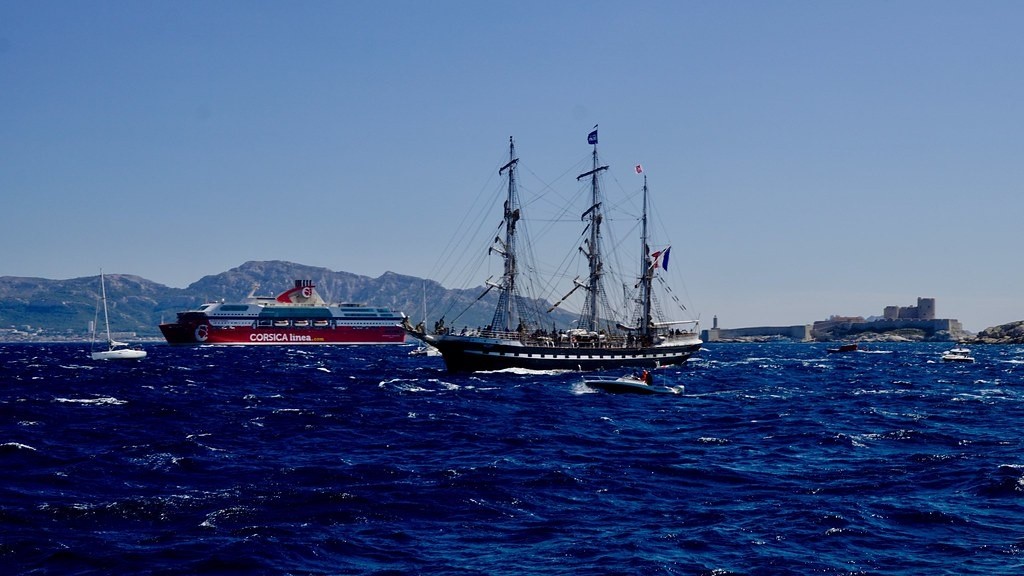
top-left (88, 269), bottom-right (147, 360)
top-left (397, 121), bottom-right (705, 372)
top-left (407, 281), bottom-right (439, 358)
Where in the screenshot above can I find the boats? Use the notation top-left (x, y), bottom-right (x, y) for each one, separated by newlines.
top-left (159, 278), bottom-right (406, 347)
top-left (826, 341), bottom-right (857, 354)
top-left (942, 348), bottom-right (975, 362)
top-left (585, 373), bottom-right (683, 397)
top-left (858, 347), bottom-right (873, 353)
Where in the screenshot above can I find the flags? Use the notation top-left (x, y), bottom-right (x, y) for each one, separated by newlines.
top-left (652, 246), bottom-right (671, 271)
top-left (637, 165), bottom-right (642, 173)
top-left (587, 130), bottom-right (598, 144)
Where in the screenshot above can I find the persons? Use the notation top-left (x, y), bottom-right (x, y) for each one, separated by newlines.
top-left (505, 324), bottom-right (563, 341)
top-left (633, 368), bottom-right (653, 386)
top-left (451, 324), bottom-right (487, 337)
top-left (671, 329), bottom-right (674, 335)
